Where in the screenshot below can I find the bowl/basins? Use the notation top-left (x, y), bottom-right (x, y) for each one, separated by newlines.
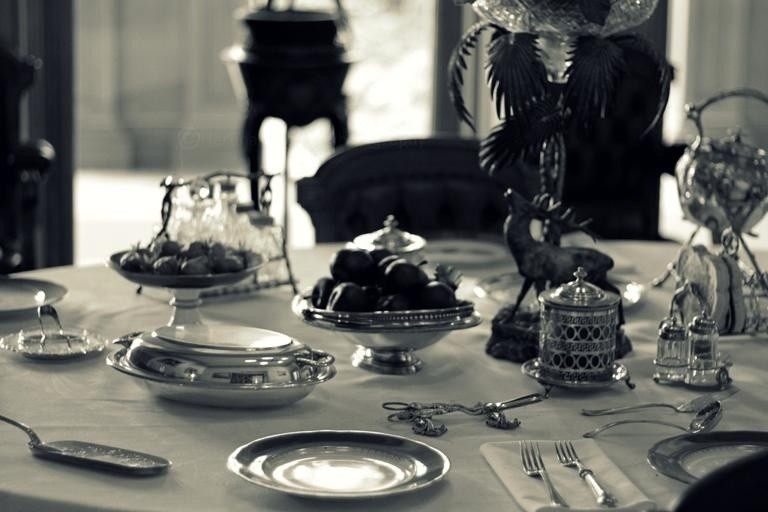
top-left (289, 290), bottom-right (484, 377)
top-left (108, 251), bottom-right (268, 325)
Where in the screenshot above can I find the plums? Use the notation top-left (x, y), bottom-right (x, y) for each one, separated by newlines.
top-left (303, 247), bottom-right (456, 313)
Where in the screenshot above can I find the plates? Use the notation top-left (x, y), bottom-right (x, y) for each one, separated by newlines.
top-left (0, 323), bottom-right (109, 363)
top-left (644, 430), bottom-right (768, 484)
top-left (104, 350), bottom-right (335, 410)
top-left (226, 430), bottom-right (451, 499)
top-left (0, 278), bottom-right (68, 320)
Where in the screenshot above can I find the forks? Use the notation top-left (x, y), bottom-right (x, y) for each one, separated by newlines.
top-left (519, 439), bottom-right (617, 509)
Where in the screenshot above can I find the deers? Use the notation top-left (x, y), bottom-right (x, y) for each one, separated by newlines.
top-left (502, 188), bottom-right (625, 330)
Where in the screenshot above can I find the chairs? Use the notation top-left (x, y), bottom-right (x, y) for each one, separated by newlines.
top-left (296, 136), bottom-right (549, 243)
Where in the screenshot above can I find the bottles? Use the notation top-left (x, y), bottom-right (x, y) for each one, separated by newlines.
top-left (656, 310), bottom-right (720, 388)
